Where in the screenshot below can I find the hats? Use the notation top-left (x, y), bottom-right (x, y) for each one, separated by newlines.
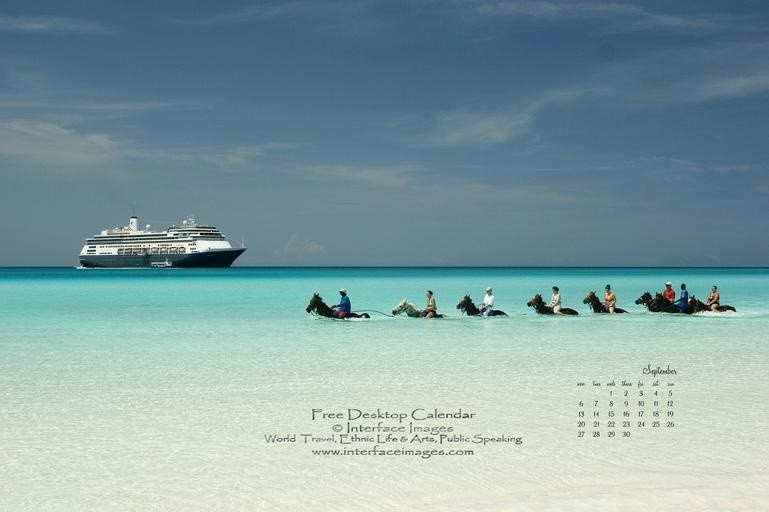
top-left (339, 289), bottom-right (347, 294)
top-left (666, 282), bottom-right (672, 285)
top-left (486, 287), bottom-right (492, 291)
top-left (605, 285), bottom-right (610, 289)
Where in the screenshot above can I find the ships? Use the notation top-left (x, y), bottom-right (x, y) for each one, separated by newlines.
top-left (77, 216), bottom-right (248, 269)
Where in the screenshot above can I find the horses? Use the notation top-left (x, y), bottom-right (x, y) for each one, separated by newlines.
top-left (635, 292), bottom-right (736, 315)
top-left (391, 298), bottom-right (448, 318)
top-left (526, 294), bottom-right (579, 315)
top-left (457, 294), bottom-right (508, 316)
top-left (306, 293), bottom-right (370, 319)
top-left (582, 291), bottom-right (631, 314)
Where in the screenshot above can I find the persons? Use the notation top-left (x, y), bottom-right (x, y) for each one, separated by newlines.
top-left (603, 284), bottom-right (616, 314)
top-left (662, 281), bottom-right (675, 305)
top-left (674, 283), bottom-right (688, 314)
top-left (331, 289), bottom-right (351, 314)
top-left (705, 285), bottom-right (720, 312)
top-left (477, 287), bottom-right (494, 317)
top-left (545, 286), bottom-right (562, 315)
top-left (422, 290), bottom-right (437, 318)
